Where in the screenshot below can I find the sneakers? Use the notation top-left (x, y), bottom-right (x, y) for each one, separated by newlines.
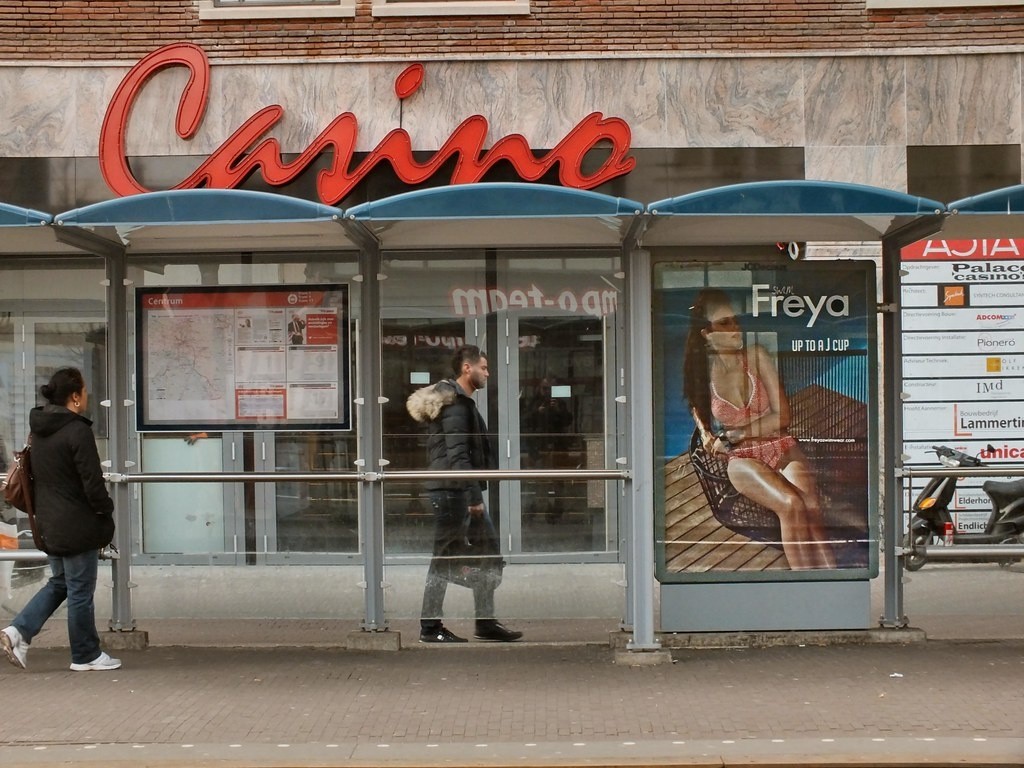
top-left (419, 627), bottom-right (469, 643)
top-left (474, 621), bottom-right (524, 641)
top-left (70, 652), bottom-right (121, 670)
top-left (0, 625), bottom-right (29, 670)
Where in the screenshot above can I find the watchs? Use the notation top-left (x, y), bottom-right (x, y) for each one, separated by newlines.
top-left (718, 429), bottom-right (733, 449)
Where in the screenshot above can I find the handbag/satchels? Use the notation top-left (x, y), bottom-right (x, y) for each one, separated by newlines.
top-left (5, 429), bottom-right (35, 516)
top-left (431, 510), bottom-right (507, 590)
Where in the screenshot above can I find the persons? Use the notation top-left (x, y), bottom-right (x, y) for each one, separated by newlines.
top-left (407, 344), bottom-right (523, 643)
top-left (521, 373), bottom-right (574, 527)
top-left (0, 365), bottom-right (122, 671)
top-left (682, 286), bottom-right (837, 570)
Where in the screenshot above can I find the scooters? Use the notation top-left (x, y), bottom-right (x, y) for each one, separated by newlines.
top-left (902, 444), bottom-right (1024, 572)
